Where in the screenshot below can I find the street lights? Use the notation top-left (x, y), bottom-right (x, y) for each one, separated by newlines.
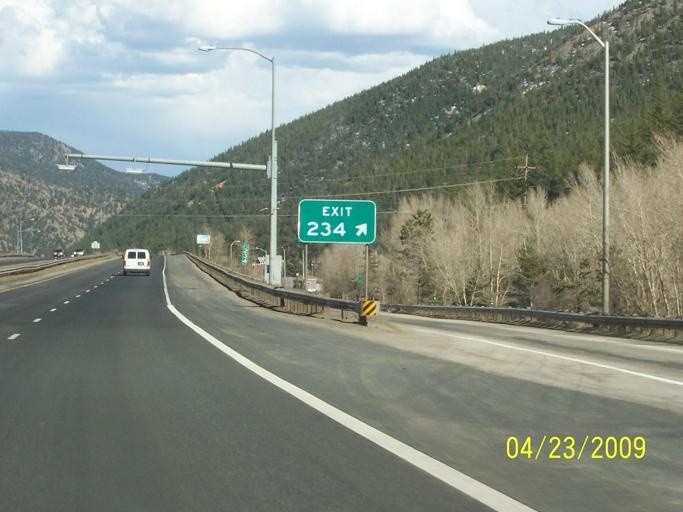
top-left (230, 240), bottom-right (240, 264)
top-left (255, 246), bottom-right (267, 257)
top-left (547, 16), bottom-right (611, 315)
top-left (198, 44), bottom-right (278, 284)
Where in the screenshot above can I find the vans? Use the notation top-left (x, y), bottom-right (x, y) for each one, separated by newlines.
top-left (121, 249), bottom-right (151, 276)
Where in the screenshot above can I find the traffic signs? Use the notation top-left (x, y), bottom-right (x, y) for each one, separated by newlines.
top-left (298, 199), bottom-right (377, 244)
